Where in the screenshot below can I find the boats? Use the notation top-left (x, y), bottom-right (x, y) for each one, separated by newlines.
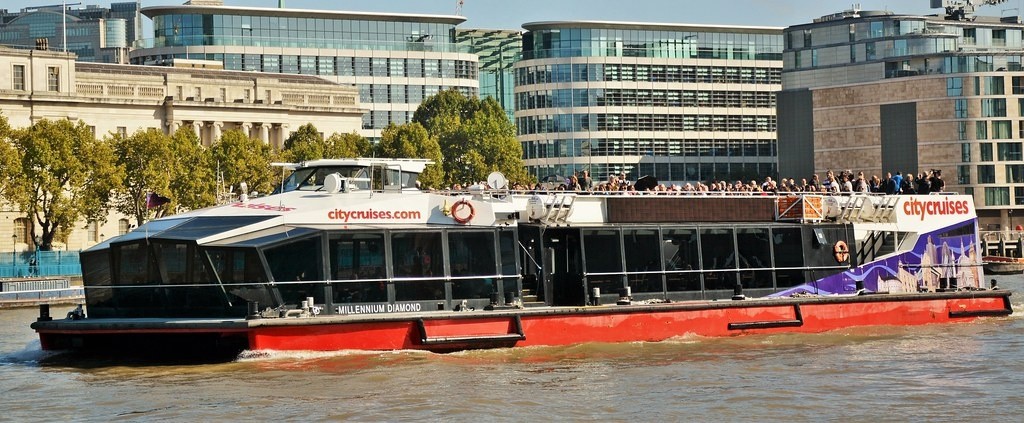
top-left (983, 256), bottom-right (1024, 275)
top-left (29, 156), bottom-right (1015, 365)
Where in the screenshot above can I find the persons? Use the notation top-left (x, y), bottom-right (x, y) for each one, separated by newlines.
top-left (854, 170), bottom-right (871, 193)
top-left (667, 180), bottom-right (751, 196)
top-left (822, 170), bottom-right (841, 196)
top-left (467, 181), bottom-right (490, 194)
top-left (620, 184), bottom-right (638, 198)
top-left (915, 169), bottom-right (946, 195)
top-left (882, 171), bottom-right (895, 193)
top-left (608, 172), bottom-right (628, 194)
top-left (580, 169), bottom-right (594, 192)
top-left (596, 183), bottom-right (612, 195)
top-left (892, 170), bottom-right (916, 194)
top-left (842, 175), bottom-right (853, 191)
top-left (510, 181), bottom-right (524, 196)
top-left (568, 174), bottom-right (581, 192)
top-left (450, 183), bottom-right (464, 195)
top-left (752, 174), bottom-right (821, 195)
top-left (869, 175), bottom-right (881, 192)
top-left (644, 183), bottom-right (666, 196)
top-left (558, 184), bottom-right (567, 191)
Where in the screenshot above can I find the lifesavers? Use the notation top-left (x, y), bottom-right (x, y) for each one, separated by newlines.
top-left (451, 200), bottom-right (475, 224)
top-left (834, 241), bottom-right (849, 262)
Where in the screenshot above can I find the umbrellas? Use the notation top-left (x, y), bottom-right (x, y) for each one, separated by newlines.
top-left (634, 174), bottom-right (658, 190)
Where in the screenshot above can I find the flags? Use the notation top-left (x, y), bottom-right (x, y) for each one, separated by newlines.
top-left (147, 192), bottom-right (171, 209)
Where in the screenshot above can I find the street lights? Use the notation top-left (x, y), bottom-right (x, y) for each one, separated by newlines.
top-left (30, 115), bottom-right (79, 251)
top-left (12, 234), bottom-right (17, 276)
